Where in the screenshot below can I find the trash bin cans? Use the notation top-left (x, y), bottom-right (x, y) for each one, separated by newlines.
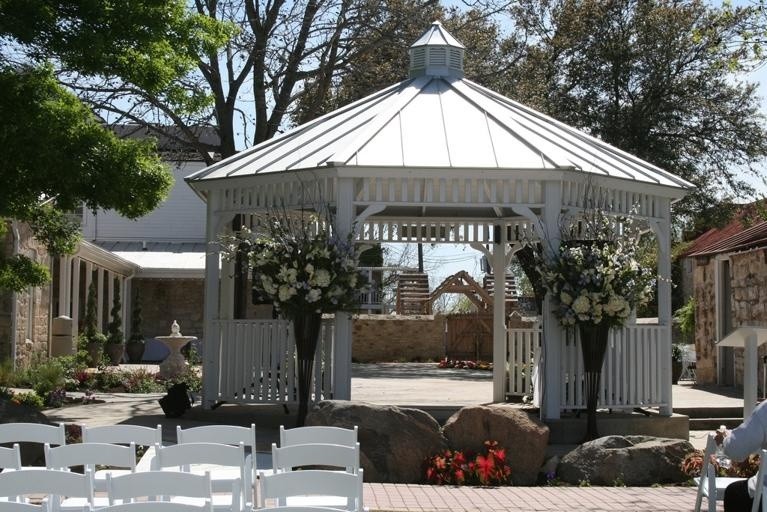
top-left (52, 315), bottom-right (74, 359)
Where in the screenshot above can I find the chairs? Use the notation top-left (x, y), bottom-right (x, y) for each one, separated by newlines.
top-left (0, 422), bottom-right (362, 512)
top-left (693, 435), bottom-right (750, 512)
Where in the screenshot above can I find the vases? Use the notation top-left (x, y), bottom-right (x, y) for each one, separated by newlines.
top-left (286, 311), bottom-right (320, 429)
top-left (579, 318), bottom-right (610, 446)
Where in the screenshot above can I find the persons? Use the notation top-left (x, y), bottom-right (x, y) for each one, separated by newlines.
top-left (723, 397), bottom-right (767, 512)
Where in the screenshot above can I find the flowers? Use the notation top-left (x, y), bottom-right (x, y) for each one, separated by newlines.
top-left (205, 169), bottom-right (412, 337)
top-left (510, 175), bottom-right (674, 334)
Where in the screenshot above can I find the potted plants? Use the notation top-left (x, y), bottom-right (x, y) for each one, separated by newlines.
top-left (671, 345), bottom-right (682, 383)
top-left (85, 278), bottom-right (145, 367)
